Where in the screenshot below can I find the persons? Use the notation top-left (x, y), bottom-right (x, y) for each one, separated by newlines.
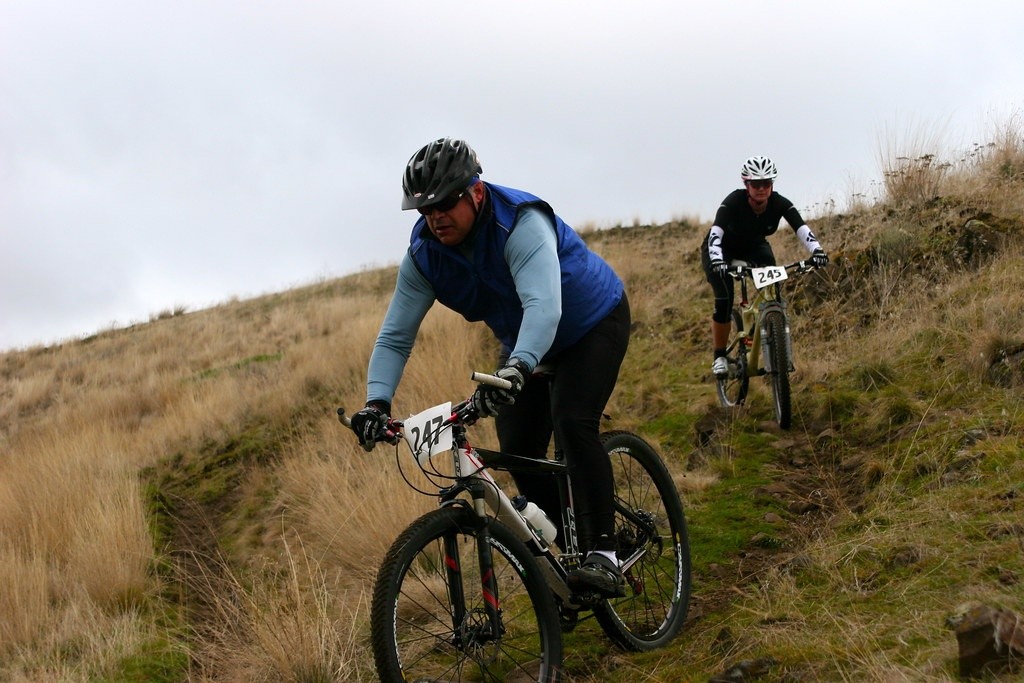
top-left (350, 138), bottom-right (632, 598)
top-left (699, 155), bottom-right (830, 375)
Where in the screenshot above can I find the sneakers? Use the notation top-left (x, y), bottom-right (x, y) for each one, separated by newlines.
top-left (711, 356), bottom-right (729, 376)
top-left (556, 594), bottom-right (581, 634)
top-left (567, 553), bottom-right (627, 597)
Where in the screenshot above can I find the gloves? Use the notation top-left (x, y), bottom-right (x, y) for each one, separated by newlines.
top-left (711, 260), bottom-right (728, 278)
top-left (812, 249), bottom-right (828, 270)
top-left (350, 404), bottom-right (394, 452)
top-left (470, 366), bottom-right (525, 419)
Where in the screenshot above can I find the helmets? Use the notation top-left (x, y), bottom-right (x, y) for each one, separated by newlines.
top-left (741, 157), bottom-right (777, 180)
top-left (402, 138), bottom-right (482, 210)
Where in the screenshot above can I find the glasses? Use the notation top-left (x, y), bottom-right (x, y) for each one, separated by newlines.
top-left (745, 180), bottom-right (773, 189)
top-left (418, 185), bottom-right (473, 216)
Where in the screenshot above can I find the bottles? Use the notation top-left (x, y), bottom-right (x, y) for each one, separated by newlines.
top-left (511, 494), bottom-right (558, 543)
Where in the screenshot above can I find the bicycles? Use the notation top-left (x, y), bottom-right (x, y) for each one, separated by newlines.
top-left (335, 367), bottom-right (692, 682)
top-left (714, 257), bottom-right (823, 430)
